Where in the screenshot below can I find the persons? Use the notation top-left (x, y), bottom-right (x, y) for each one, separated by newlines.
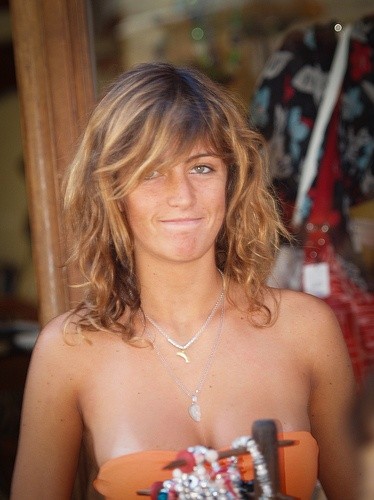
top-left (12, 65), bottom-right (362, 499)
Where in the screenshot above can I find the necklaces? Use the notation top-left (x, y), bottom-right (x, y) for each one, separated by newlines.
top-left (137, 264), bottom-right (227, 424)
